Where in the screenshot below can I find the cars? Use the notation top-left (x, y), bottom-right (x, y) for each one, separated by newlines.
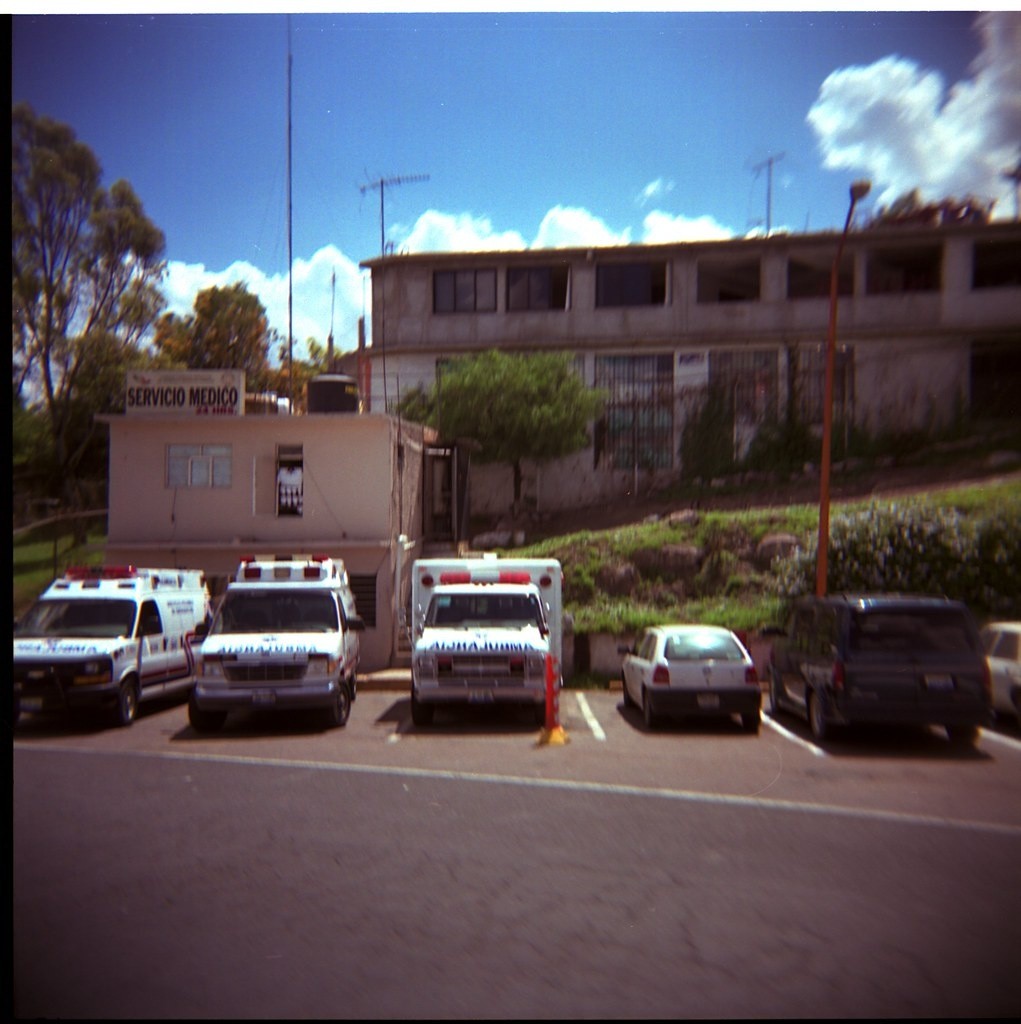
top-left (980, 620), bottom-right (1021, 731)
top-left (617, 622), bottom-right (766, 733)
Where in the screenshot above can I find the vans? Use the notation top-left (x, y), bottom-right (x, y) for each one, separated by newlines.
top-left (14, 564), bottom-right (213, 728)
top-left (179, 553), bottom-right (364, 726)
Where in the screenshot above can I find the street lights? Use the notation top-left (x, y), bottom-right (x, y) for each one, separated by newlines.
top-left (816, 178), bottom-right (870, 599)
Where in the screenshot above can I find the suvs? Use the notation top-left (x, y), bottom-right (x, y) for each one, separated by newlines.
top-left (761, 593), bottom-right (991, 753)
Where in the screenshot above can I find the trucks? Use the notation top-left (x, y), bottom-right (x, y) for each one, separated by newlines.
top-left (405, 552), bottom-right (563, 729)
